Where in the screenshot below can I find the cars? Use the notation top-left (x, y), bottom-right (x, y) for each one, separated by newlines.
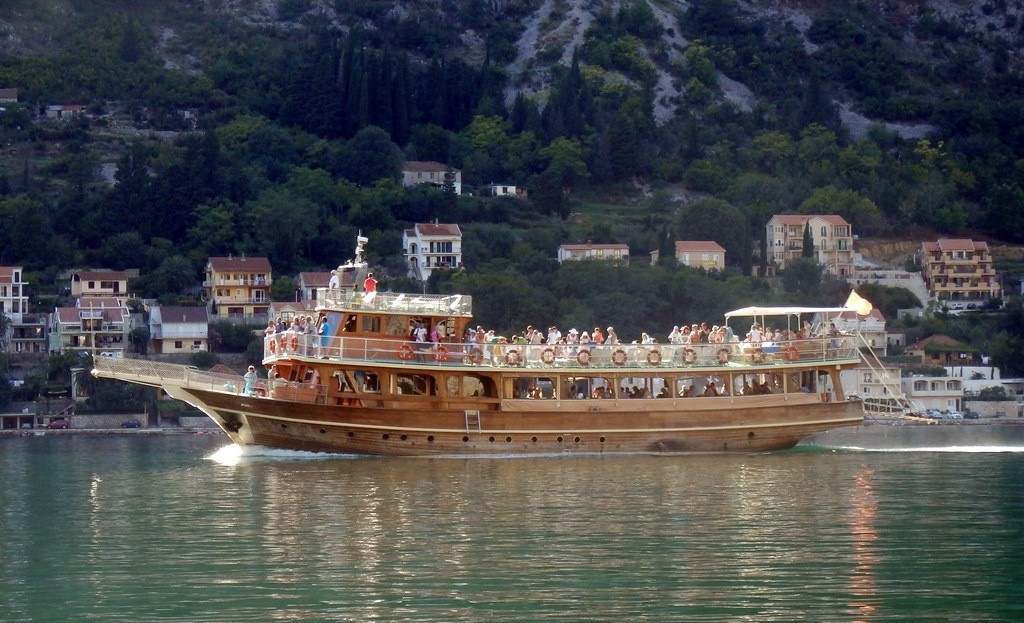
top-left (910, 408), bottom-right (943, 419)
top-left (964, 411), bottom-right (979, 419)
top-left (946, 411), bottom-right (963, 419)
top-left (47, 419), bottom-right (68, 429)
top-left (121, 419), bottom-right (141, 429)
top-left (22, 421), bottom-right (32, 429)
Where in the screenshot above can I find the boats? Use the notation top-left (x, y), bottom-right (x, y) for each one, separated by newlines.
top-left (90, 231), bottom-right (866, 465)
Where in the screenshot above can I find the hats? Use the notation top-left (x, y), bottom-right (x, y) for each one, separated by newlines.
top-left (569, 328), bottom-right (579, 334)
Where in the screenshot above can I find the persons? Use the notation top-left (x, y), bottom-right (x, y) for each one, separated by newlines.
top-left (225, 365), bottom-right (373, 405)
top-left (414, 322), bottom-right (428, 362)
top-left (263, 315), bottom-right (331, 359)
top-left (463, 325), bottom-right (619, 366)
top-left (363, 273), bottom-right (378, 293)
top-left (429, 324), bottom-right (444, 364)
top-left (328, 270), bottom-right (339, 290)
top-left (637, 321), bottom-right (841, 359)
top-left (513, 384), bottom-right (814, 399)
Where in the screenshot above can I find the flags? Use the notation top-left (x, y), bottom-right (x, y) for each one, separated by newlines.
top-left (845, 291), bottom-right (873, 315)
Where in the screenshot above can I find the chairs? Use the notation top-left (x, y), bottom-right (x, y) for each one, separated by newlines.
top-left (525, 346), bottom-right (729, 369)
top-left (359, 291), bottom-right (463, 314)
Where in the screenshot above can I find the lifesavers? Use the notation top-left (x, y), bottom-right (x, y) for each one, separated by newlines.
top-left (505, 349), bottom-right (521, 365)
top-left (279, 336), bottom-right (287, 350)
top-left (786, 346), bottom-right (800, 361)
top-left (540, 348), bottom-right (556, 365)
top-left (716, 348), bottom-right (731, 364)
top-left (470, 348), bottom-right (483, 363)
top-left (290, 336), bottom-right (298, 351)
top-left (647, 350), bottom-right (662, 365)
top-left (398, 344), bottom-right (414, 360)
top-left (682, 349), bottom-right (696, 364)
top-left (612, 349), bottom-right (627, 365)
top-left (751, 348), bottom-right (765, 363)
top-left (269, 338), bottom-right (275, 354)
top-left (433, 346), bottom-right (449, 362)
top-left (577, 350), bottom-right (591, 365)
